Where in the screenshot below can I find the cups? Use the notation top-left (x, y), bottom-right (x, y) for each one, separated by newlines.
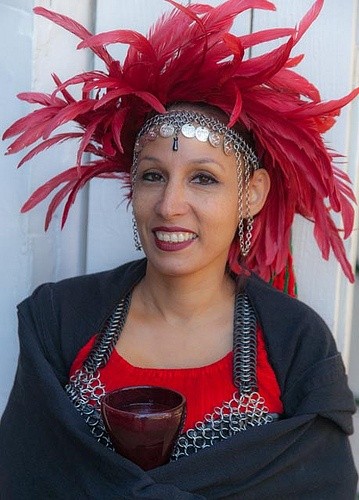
top-left (98, 385), bottom-right (185, 473)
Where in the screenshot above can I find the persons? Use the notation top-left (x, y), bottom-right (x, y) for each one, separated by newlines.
top-left (0, 98), bottom-right (359, 500)
